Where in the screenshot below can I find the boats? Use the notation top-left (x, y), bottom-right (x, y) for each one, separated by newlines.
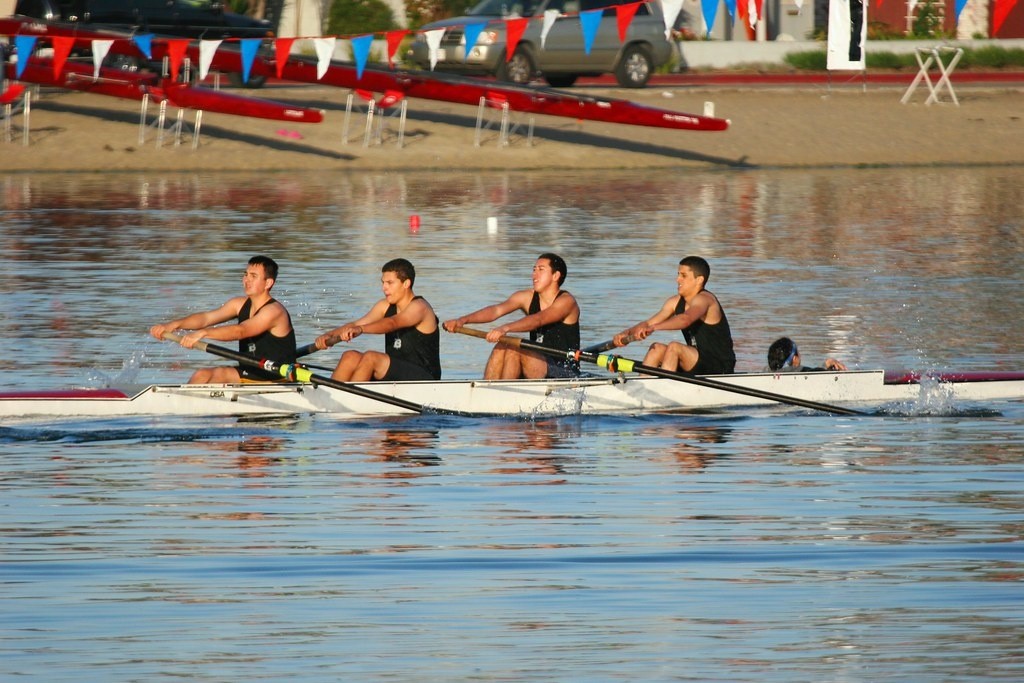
top-left (0, 325), bottom-right (1023, 427)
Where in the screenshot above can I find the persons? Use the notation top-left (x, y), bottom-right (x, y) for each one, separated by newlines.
top-left (312, 258), bottom-right (442, 383)
top-left (442, 253), bottom-right (581, 380)
top-left (768, 337), bottom-right (847, 373)
top-left (611, 256), bottom-right (738, 376)
top-left (150, 255), bottom-right (298, 385)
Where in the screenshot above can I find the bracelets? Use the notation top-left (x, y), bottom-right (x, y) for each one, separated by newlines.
top-left (357, 326), bottom-right (363, 333)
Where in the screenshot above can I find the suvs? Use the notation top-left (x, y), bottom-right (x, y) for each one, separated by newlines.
top-left (8, 0), bottom-right (273, 91)
top-left (413, 0), bottom-right (671, 89)
